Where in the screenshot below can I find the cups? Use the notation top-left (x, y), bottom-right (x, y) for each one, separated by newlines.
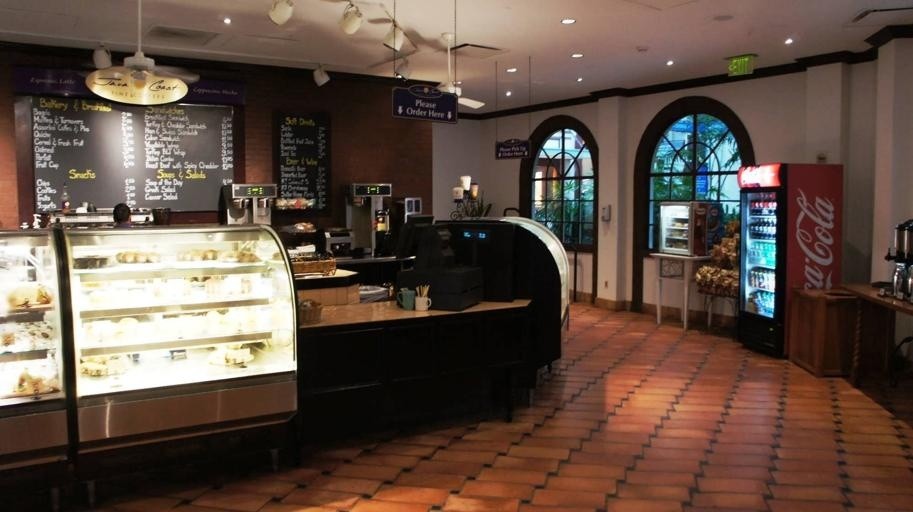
top-left (396, 290), bottom-right (415, 311)
top-left (414, 297), bottom-right (430, 312)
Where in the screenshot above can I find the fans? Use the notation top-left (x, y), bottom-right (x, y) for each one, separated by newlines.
top-left (76, 2), bottom-right (204, 88)
top-left (421, 32), bottom-right (486, 111)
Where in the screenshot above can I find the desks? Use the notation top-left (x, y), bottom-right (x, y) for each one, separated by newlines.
top-left (841, 279), bottom-right (913, 394)
top-left (695, 288), bottom-right (741, 335)
top-left (649, 252), bottom-right (718, 332)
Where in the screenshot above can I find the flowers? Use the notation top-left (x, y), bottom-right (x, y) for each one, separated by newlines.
top-left (695, 220), bottom-right (740, 293)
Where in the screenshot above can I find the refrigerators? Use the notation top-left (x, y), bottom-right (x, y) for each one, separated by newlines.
top-left (734, 163), bottom-right (843, 357)
top-left (658, 202), bottom-right (722, 258)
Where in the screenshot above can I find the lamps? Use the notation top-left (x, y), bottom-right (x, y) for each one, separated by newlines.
top-left (451, 174), bottom-right (480, 206)
top-left (267, 0), bottom-right (420, 83)
top-left (312, 65), bottom-right (330, 88)
top-left (90, 38), bottom-right (113, 72)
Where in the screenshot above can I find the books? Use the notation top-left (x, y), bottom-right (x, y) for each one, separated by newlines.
top-left (361, 282), bottom-right (391, 306)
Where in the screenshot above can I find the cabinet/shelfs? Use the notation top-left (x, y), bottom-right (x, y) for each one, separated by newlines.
top-left (786, 287), bottom-right (897, 378)
top-left (0, 229), bottom-right (72, 470)
top-left (435, 213), bottom-right (573, 376)
top-left (55, 216), bottom-right (298, 453)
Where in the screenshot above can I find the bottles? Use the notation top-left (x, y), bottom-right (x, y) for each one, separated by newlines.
top-left (60, 182), bottom-right (71, 215)
top-left (744, 192), bottom-right (776, 312)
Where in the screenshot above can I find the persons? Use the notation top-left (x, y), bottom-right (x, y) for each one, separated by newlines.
top-left (110, 200), bottom-right (138, 229)
